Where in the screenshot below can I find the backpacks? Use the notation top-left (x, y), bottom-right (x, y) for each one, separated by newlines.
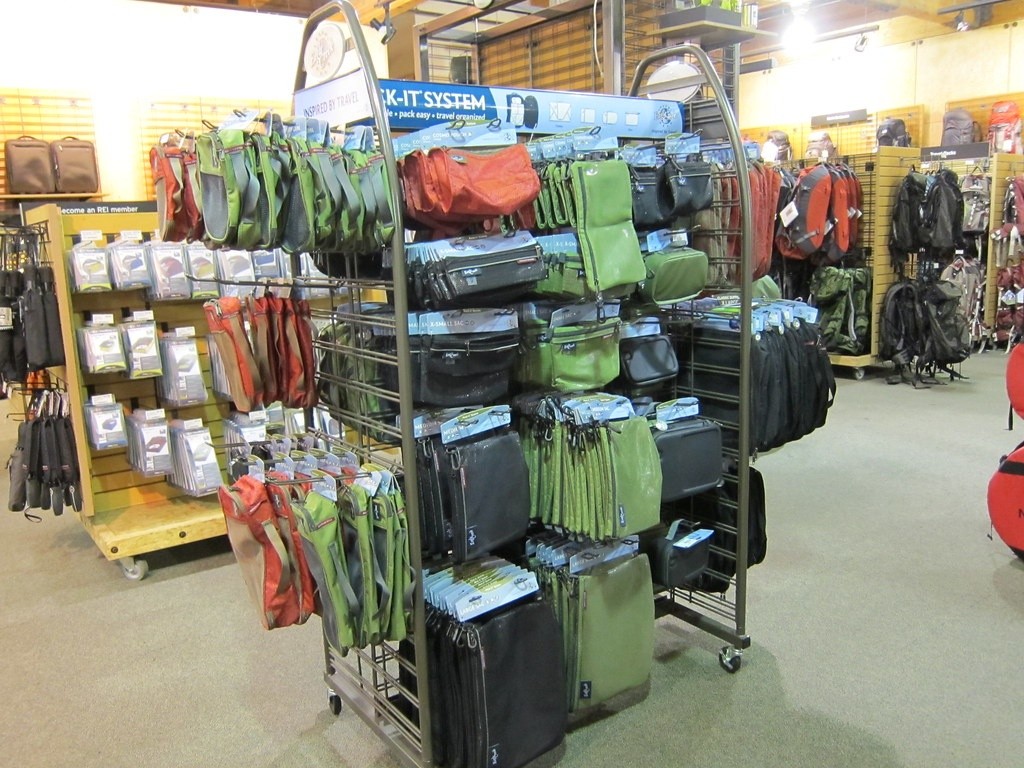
top-left (986, 100), bottom-right (1023, 158)
top-left (770, 161), bottom-right (1024, 389)
top-left (4, 136), bottom-right (98, 194)
top-left (761, 130), bottom-right (793, 160)
top-left (876, 116), bottom-right (911, 148)
top-left (941, 107), bottom-right (983, 146)
top-left (804, 131), bottom-right (837, 158)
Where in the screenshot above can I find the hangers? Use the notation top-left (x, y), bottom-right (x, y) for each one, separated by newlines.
top-left (669, 297), bottom-right (818, 337)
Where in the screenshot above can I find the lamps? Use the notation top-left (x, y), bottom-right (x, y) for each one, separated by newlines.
top-left (370, 3), bottom-right (397, 44)
top-left (855, 33), bottom-right (870, 52)
top-left (953, 10), bottom-right (969, 32)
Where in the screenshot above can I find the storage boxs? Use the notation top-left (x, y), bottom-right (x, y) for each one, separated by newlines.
top-left (658, 6), bottom-right (742, 29)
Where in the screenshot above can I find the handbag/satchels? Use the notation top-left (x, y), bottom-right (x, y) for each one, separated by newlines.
top-left (203, 295), bottom-right (319, 413)
top-left (147, 130), bottom-right (393, 255)
top-left (318, 143), bottom-right (836, 768)
top-left (218, 441), bottom-right (408, 657)
